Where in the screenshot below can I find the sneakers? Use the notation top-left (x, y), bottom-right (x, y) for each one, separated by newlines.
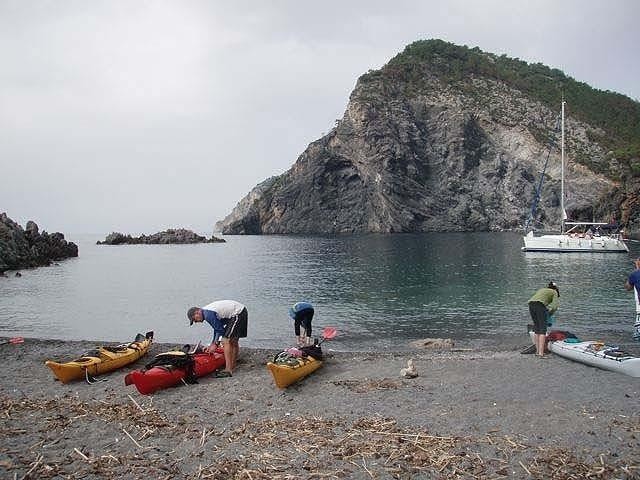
top-left (535, 352), bottom-right (546, 356)
top-left (216, 371), bottom-right (233, 378)
top-left (538, 355), bottom-right (549, 359)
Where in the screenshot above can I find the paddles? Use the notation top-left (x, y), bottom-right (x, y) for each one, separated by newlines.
top-left (317, 327), bottom-right (337, 348)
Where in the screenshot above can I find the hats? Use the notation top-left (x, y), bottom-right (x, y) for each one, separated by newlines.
top-left (551, 283), bottom-right (559, 297)
top-left (187, 306), bottom-right (197, 326)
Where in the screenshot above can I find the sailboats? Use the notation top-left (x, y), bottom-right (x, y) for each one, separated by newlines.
top-left (520, 85), bottom-right (629, 253)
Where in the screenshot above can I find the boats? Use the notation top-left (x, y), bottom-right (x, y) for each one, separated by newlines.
top-left (124, 345), bottom-right (225, 394)
top-left (266, 338), bottom-right (324, 389)
top-left (527, 326), bottom-right (640, 377)
top-left (45, 330), bottom-right (155, 385)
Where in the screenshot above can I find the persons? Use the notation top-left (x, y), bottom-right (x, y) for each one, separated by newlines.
top-left (288, 302), bottom-right (314, 346)
top-left (624, 257), bottom-right (640, 340)
top-left (526, 282), bottom-right (560, 359)
top-left (187, 300), bottom-right (248, 377)
